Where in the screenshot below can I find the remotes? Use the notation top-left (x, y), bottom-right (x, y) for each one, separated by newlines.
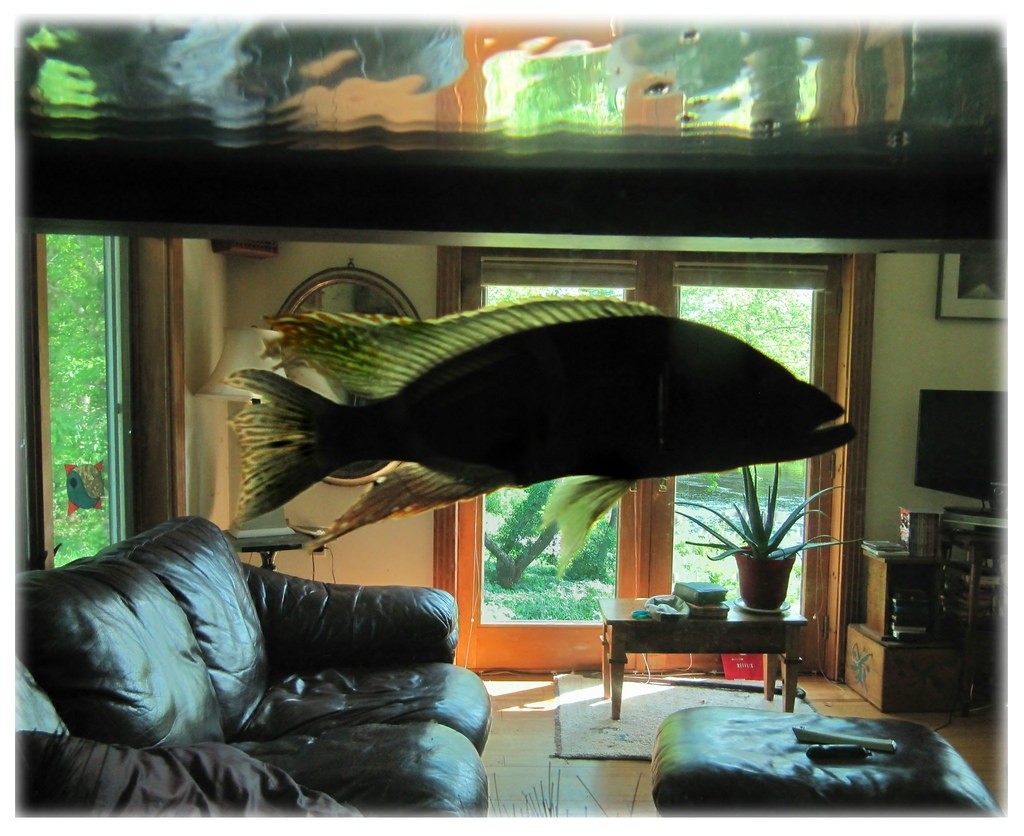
top-left (792, 726), bottom-right (898, 758)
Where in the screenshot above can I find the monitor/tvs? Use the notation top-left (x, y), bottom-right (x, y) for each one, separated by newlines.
top-left (913, 387), bottom-right (1009, 519)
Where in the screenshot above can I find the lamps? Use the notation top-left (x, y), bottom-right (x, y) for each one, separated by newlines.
top-left (193, 327), bottom-right (295, 537)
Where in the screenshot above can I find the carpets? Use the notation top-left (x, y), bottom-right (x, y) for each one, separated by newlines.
top-left (554, 672), bottom-right (818, 759)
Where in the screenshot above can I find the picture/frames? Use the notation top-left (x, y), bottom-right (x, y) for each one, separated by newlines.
top-left (934, 252), bottom-right (1004, 321)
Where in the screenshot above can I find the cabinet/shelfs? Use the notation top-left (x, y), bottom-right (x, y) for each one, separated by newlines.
top-left (862, 549), bottom-right (957, 649)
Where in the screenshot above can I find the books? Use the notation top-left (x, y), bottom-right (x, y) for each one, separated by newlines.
top-left (686, 601), bottom-right (730, 619)
top-left (673, 581), bottom-right (728, 604)
top-left (862, 507), bottom-right (1007, 644)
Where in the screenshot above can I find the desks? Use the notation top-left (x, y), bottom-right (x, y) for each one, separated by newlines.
top-left (222, 526), bottom-right (326, 571)
top-left (940, 524), bottom-right (1004, 717)
top-left (598, 596), bottom-right (808, 720)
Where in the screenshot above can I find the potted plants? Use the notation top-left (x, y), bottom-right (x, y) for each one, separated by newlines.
top-left (667, 462), bottom-right (870, 609)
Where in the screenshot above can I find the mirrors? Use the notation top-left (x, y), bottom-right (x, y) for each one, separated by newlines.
top-left (267, 268), bottom-right (423, 487)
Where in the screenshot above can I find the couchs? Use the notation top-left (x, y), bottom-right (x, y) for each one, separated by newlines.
top-left (17, 516), bottom-right (493, 817)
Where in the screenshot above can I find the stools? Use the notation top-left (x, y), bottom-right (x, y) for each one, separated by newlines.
top-left (650, 706), bottom-right (1004, 817)
